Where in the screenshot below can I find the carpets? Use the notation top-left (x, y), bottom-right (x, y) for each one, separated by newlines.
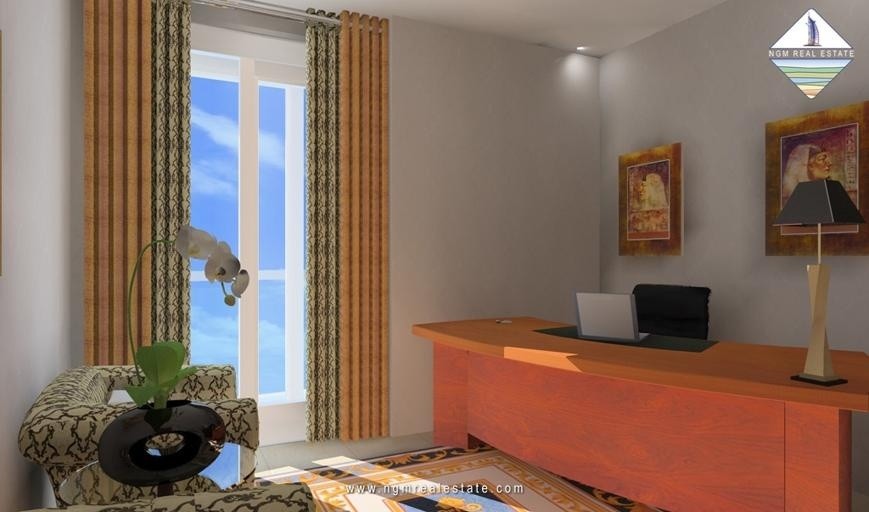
top-left (259, 439), bottom-right (666, 512)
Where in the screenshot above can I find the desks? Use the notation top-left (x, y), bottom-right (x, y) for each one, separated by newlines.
top-left (411, 313), bottom-right (869, 512)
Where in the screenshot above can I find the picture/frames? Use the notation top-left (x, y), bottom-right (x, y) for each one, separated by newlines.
top-left (617, 141), bottom-right (686, 259)
top-left (764, 99), bottom-right (869, 258)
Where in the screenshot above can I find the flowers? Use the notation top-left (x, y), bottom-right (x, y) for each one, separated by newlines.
top-left (124, 224), bottom-right (250, 409)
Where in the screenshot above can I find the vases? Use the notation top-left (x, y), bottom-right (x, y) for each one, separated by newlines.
top-left (97, 398), bottom-right (227, 487)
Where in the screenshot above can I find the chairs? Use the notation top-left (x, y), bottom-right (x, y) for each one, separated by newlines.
top-left (18, 362), bottom-right (260, 507)
top-left (20, 480), bottom-right (315, 512)
top-left (632, 284), bottom-right (712, 341)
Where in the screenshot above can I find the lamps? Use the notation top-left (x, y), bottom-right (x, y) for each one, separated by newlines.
top-left (772, 179), bottom-right (866, 387)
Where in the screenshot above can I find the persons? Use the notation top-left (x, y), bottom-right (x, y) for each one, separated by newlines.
top-left (782, 141), bottom-right (833, 199)
top-left (638, 174), bottom-right (669, 209)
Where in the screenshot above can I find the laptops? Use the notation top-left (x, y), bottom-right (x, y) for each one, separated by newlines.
top-left (574, 289), bottom-right (651, 342)
